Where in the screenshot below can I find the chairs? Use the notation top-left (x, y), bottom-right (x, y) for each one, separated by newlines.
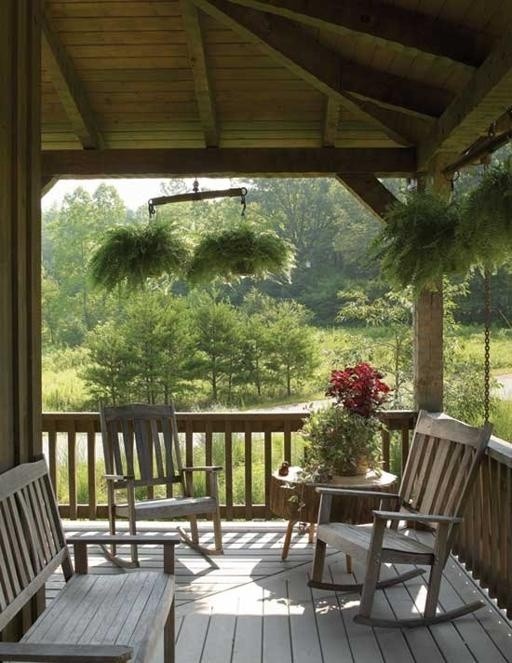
top-left (309, 408), bottom-right (496, 629)
top-left (97, 401), bottom-right (233, 570)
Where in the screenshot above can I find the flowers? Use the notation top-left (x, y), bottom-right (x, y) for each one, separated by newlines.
top-left (323, 361), bottom-right (394, 418)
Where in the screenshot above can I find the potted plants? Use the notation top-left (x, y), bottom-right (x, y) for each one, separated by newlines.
top-left (81, 214), bottom-right (192, 290)
top-left (286, 406), bottom-right (388, 535)
top-left (181, 225), bottom-right (297, 287)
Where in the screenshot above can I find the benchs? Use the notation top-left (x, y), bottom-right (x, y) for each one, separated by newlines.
top-left (1, 456), bottom-right (184, 662)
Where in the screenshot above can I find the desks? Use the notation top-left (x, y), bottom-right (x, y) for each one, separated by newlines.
top-left (267, 460), bottom-right (401, 576)
